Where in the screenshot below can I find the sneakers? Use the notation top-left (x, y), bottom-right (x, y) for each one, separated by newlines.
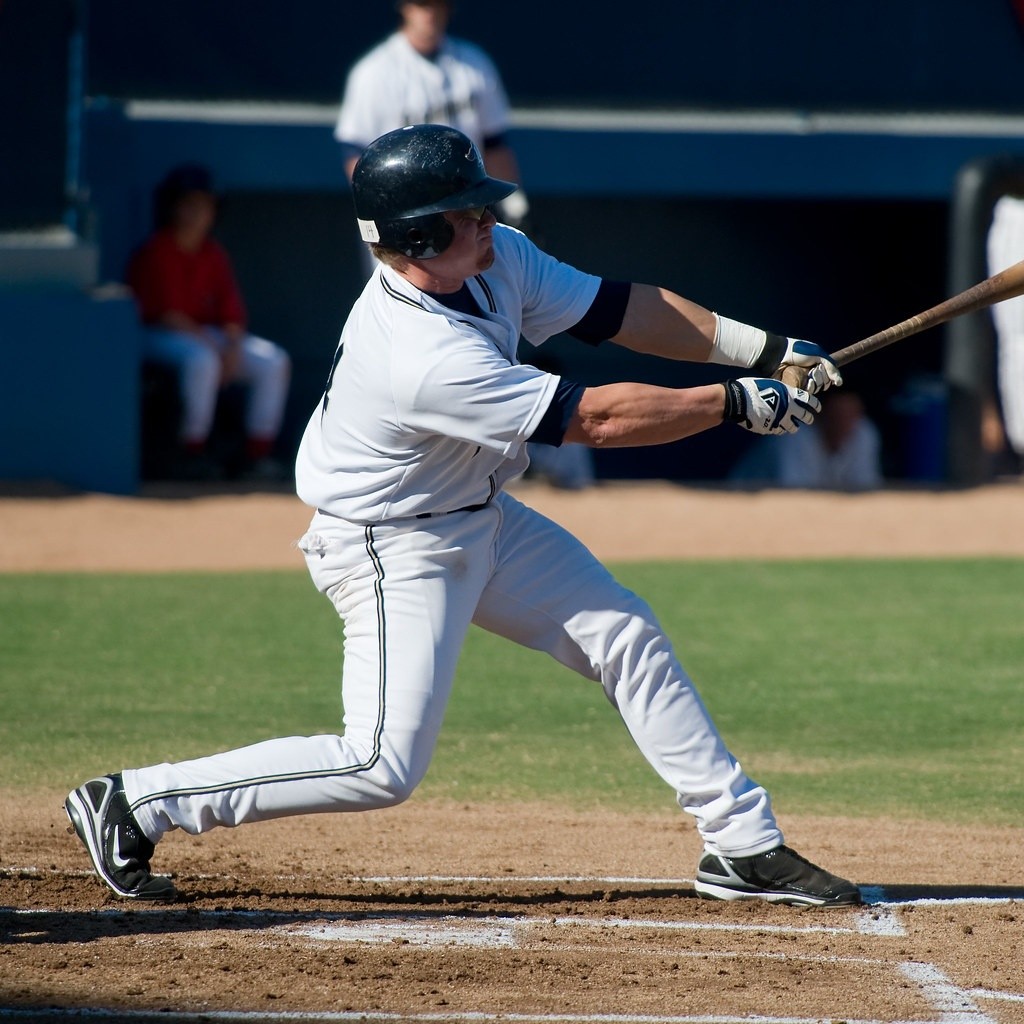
top-left (694, 844), bottom-right (861, 908)
top-left (64, 772), bottom-right (178, 904)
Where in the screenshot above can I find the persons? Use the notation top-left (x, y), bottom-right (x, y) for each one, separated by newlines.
top-left (757, 376), bottom-right (885, 492)
top-left (62, 123), bottom-right (860, 905)
top-left (126, 160), bottom-right (291, 484)
top-left (337, 0), bottom-right (529, 236)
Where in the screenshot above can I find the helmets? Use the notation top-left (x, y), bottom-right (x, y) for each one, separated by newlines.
top-left (352, 124), bottom-right (517, 261)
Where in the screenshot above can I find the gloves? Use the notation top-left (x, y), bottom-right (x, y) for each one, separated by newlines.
top-left (746, 330), bottom-right (843, 395)
top-left (719, 377), bottom-right (822, 436)
top-left (499, 191), bottom-right (528, 221)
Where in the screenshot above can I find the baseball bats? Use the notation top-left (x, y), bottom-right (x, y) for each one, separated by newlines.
top-left (779, 259), bottom-right (1024, 392)
